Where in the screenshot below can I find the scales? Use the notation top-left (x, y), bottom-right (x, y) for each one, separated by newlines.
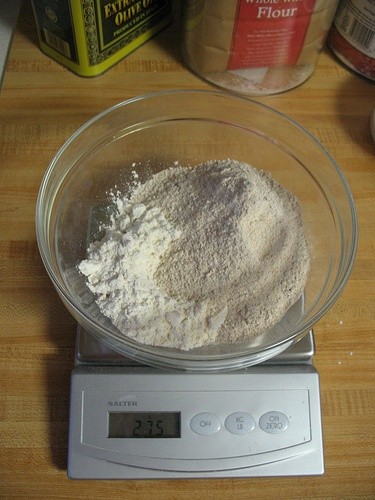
top-left (34, 87), bottom-right (363, 481)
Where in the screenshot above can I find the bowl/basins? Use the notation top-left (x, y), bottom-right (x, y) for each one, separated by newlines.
top-left (37, 89), bottom-right (356, 374)
